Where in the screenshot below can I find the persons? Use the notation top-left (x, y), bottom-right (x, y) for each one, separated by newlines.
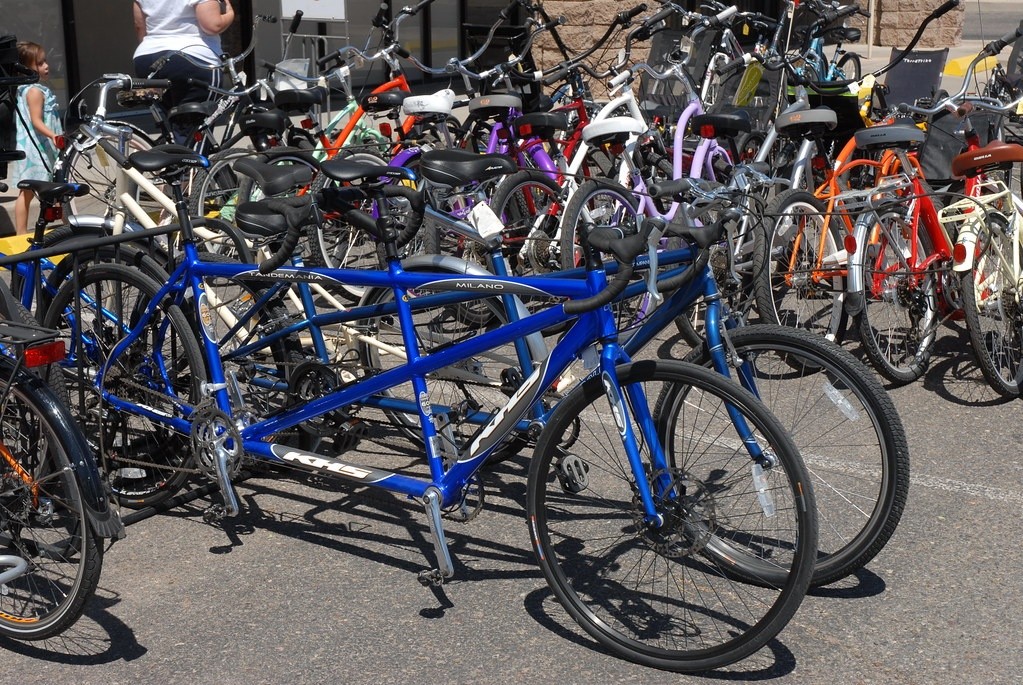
top-left (134, 0), bottom-right (235, 178)
top-left (15, 41), bottom-right (64, 236)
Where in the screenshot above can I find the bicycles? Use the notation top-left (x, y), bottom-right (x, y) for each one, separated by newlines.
top-left (0, 0), bottom-right (1023, 676)
